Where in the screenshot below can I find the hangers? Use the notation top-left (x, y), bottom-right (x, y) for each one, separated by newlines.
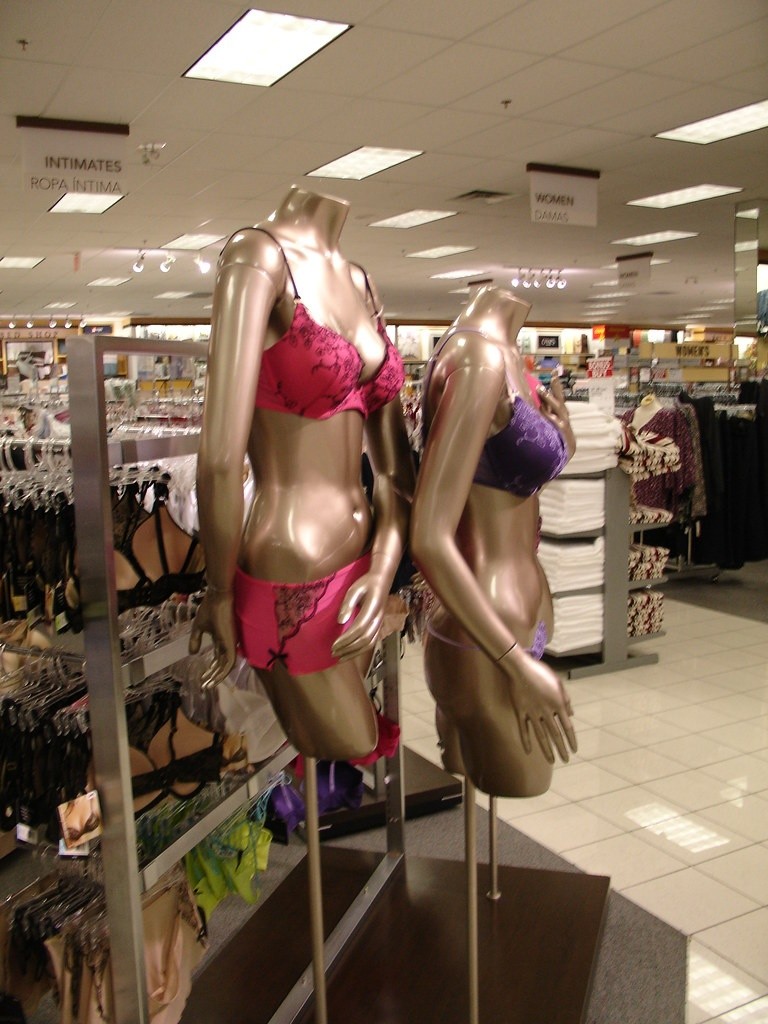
top-left (562, 385), bottom-right (758, 420)
top-left (0, 398), bottom-right (204, 514)
top-left (0, 594), bottom-right (236, 931)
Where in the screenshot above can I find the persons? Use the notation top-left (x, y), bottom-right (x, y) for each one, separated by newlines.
top-left (187, 185), bottom-right (409, 762)
top-left (412, 287), bottom-right (579, 799)
top-left (621, 394), bottom-right (696, 509)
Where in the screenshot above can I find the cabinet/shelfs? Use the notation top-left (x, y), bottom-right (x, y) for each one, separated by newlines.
top-left (539, 465), bottom-right (670, 680)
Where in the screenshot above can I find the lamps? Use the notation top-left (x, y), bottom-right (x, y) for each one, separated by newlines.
top-left (510, 268), bottom-right (566, 290)
top-left (193, 256), bottom-right (211, 274)
top-left (161, 249), bottom-right (176, 273)
top-left (133, 249), bottom-right (145, 272)
